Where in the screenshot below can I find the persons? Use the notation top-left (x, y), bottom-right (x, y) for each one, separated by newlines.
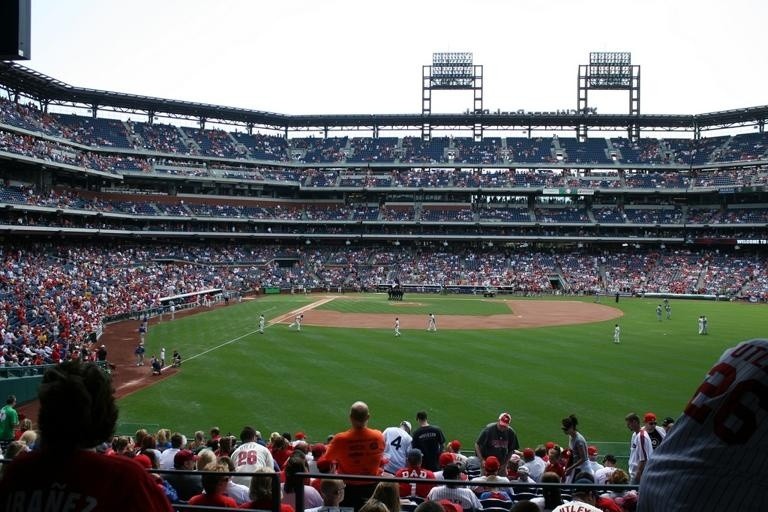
top-left (698, 314), bottom-right (708, 335)
top-left (656, 304), bottom-right (663, 321)
top-left (393, 317), bottom-right (402, 336)
top-left (258, 314), bottom-right (265, 334)
top-left (613, 324), bottom-right (621, 343)
top-left (0, 361), bottom-right (173, 511)
top-left (0, 241), bottom-right (258, 378)
top-left (665, 304), bottom-right (672, 320)
top-left (0, 394), bottom-right (38, 460)
top-left (637, 336), bottom-right (768, 512)
top-left (94, 400), bottom-right (673, 511)
top-left (426, 313), bottom-right (437, 332)
top-left (654, 244), bottom-right (767, 307)
top-left (259, 242), bottom-right (655, 305)
top-left (289, 314), bottom-right (304, 331)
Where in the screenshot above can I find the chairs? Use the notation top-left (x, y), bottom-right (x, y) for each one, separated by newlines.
top-left (399, 451), bottom-right (614, 512)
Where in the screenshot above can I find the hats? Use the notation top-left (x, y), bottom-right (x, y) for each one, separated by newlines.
top-left (131, 412), bottom-right (657, 478)
